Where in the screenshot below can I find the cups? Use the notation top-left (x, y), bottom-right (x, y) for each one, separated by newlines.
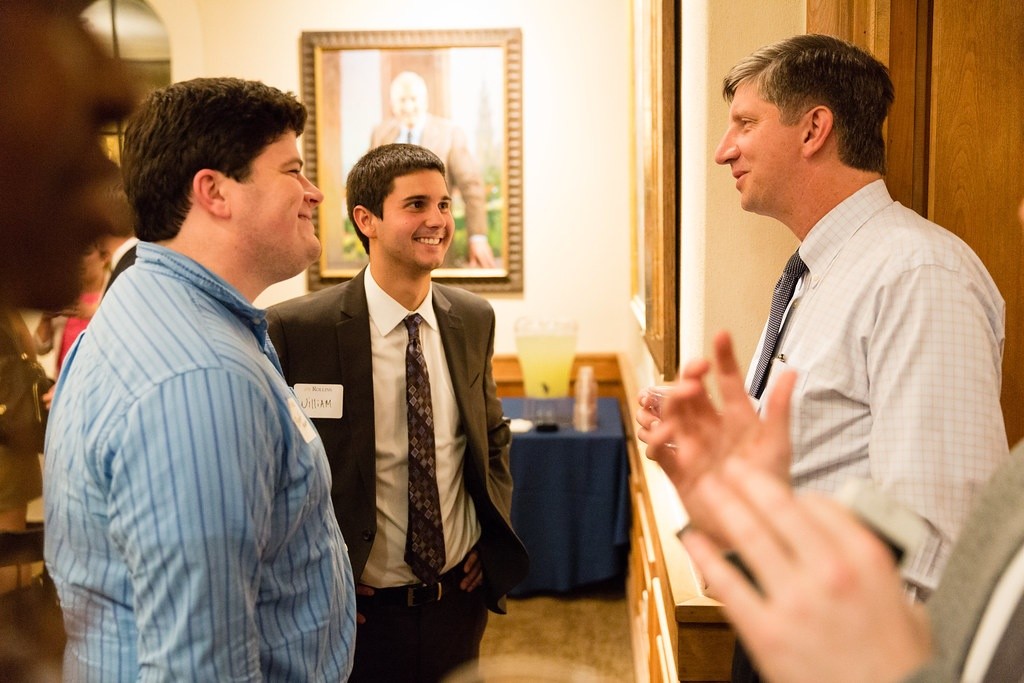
top-left (646, 384), bottom-right (712, 449)
top-left (535, 407), bottom-right (560, 433)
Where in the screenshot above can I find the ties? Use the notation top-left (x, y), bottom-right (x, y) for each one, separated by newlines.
top-left (749, 249), bottom-right (806, 399)
top-left (403, 313), bottom-right (446, 586)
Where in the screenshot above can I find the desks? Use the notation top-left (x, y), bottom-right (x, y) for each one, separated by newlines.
top-left (500, 396), bottom-right (628, 600)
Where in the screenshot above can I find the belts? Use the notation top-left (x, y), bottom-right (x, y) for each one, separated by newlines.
top-left (356, 567), bottom-right (465, 608)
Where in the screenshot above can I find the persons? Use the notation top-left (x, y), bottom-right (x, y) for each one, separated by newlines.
top-left (42, 77), bottom-right (356, 683)
top-left (0, 0), bottom-right (130, 309)
top-left (646, 330), bottom-right (1024, 683)
top-left (635, 35), bottom-right (1009, 683)
top-left (372, 70), bottom-right (495, 267)
top-left (264, 143), bottom-right (534, 683)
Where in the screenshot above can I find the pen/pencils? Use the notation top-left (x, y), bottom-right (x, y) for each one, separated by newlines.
top-left (779, 353), bottom-right (785, 363)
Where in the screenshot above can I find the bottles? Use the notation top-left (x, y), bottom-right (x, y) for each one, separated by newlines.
top-left (572, 365), bottom-right (599, 432)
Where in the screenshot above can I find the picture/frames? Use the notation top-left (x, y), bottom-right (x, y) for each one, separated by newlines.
top-left (298, 27), bottom-right (524, 295)
top-left (625, 0), bottom-right (679, 384)
